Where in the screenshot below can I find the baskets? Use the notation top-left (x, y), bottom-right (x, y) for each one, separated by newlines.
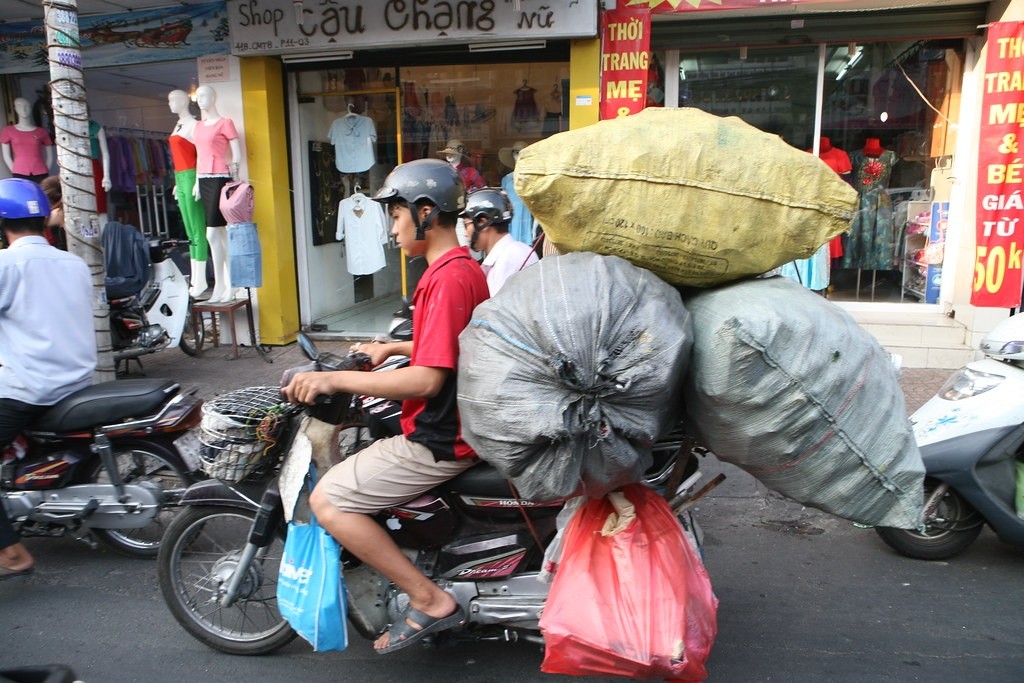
top-left (196, 385), bottom-right (302, 482)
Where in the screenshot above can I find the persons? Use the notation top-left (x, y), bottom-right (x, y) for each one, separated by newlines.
top-left (498, 141), bottom-right (539, 246)
top-left (88, 120), bottom-right (112, 230)
top-left (194, 85), bottom-right (240, 303)
top-left (805, 136), bottom-right (852, 258)
top-left (435, 139), bottom-right (488, 193)
top-left (168, 89), bottom-right (208, 296)
top-left (842, 138), bottom-right (899, 300)
top-left (41, 177), bottom-right (68, 250)
top-left (459, 189), bottom-right (538, 299)
top-left (1, 178), bottom-right (97, 582)
top-left (220, 179), bottom-right (273, 363)
top-left (280, 159), bottom-right (492, 656)
top-left (0, 98), bottom-right (52, 183)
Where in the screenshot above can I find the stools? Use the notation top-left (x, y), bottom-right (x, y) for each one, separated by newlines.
top-left (191, 299), bottom-right (253, 358)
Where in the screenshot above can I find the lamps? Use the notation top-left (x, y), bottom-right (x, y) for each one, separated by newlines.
top-left (281, 51), bottom-right (353, 63)
top-left (468, 40), bottom-right (546, 53)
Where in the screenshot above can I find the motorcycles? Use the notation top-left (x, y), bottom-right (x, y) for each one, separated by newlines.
top-left (105, 236), bottom-right (206, 376)
top-left (155, 327), bottom-right (717, 656)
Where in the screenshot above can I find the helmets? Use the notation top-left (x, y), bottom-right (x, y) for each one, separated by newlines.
top-left (458, 187), bottom-right (515, 221)
top-left (0, 178), bottom-right (50, 217)
top-left (372, 158), bottom-right (469, 209)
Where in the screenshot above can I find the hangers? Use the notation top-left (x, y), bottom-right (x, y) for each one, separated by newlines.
top-left (103, 126), bottom-right (170, 140)
top-left (519, 80), bottom-right (534, 91)
top-left (350, 184), bottom-right (366, 210)
top-left (345, 103), bottom-right (359, 118)
top-left (551, 84), bottom-right (560, 93)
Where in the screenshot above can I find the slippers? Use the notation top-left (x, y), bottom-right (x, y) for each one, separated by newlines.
top-left (376, 595), bottom-right (465, 655)
top-left (0, 563), bottom-right (35, 581)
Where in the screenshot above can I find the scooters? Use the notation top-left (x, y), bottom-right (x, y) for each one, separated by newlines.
top-left (1, 377), bottom-right (206, 558)
top-left (877, 308), bottom-right (1024, 561)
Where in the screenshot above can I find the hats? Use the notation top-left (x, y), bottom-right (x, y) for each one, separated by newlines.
top-left (498, 141), bottom-right (530, 167)
top-left (435, 139), bottom-right (474, 160)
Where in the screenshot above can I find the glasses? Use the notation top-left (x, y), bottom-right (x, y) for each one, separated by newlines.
top-left (464, 222), bottom-right (473, 230)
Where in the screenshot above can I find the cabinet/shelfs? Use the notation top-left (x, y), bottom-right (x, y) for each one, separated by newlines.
top-left (901, 201), bottom-right (949, 303)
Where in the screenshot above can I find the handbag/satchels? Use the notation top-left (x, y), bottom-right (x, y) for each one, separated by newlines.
top-left (275, 464), bottom-right (349, 652)
top-left (536, 482), bottom-right (719, 683)
